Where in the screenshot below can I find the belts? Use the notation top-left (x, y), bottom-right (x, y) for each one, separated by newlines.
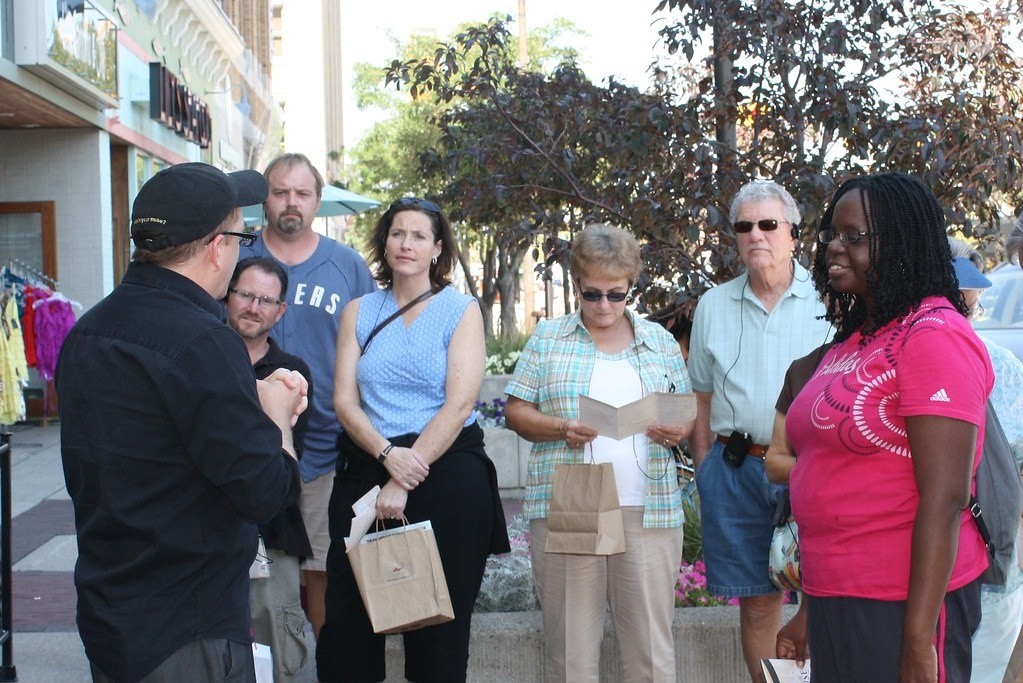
top-left (716, 434), bottom-right (769, 459)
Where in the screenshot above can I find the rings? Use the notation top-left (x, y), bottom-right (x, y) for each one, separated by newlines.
top-left (575, 443), bottom-right (579, 447)
top-left (662, 439), bottom-right (672, 447)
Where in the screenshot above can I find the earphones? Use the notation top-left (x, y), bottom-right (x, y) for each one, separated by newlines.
top-left (791, 223), bottom-right (800, 239)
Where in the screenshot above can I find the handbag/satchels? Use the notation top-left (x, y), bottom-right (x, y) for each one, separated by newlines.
top-left (766, 488), bottom-right (804, 593)
top-left (544, 440), bottom-right (627, 555)
top-left (959, 399), bottom-right (1022, 586)
top-left (348, 513), bottom-right (456, 631)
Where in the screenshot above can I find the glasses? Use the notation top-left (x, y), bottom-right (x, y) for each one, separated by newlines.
top-left (818, 228), bottom-right (877, 244)
top-left (229, 287), bottom-right (282, 309)
top-left (204, 228), bottom-right (258, 247)
top-left (733, 219), bottom-right (791, 234)
top-left (966, 300), bottom-right (985, 322)
top-left (578, 279), bottom-right (630, 302)
top-left (388, 198), bottom-right (440, 221)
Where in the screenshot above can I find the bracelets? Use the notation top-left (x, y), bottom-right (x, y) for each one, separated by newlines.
top-left (376, 443), bottom-right (394, 463)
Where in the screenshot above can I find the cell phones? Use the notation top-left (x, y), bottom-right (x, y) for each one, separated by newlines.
top-left (772, 488), bottom-right (790, 526)
top-left (721, 431), bottom-right (752, 467)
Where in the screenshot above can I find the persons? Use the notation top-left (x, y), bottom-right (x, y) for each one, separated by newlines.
top-left (783, 172), bottom-right (996, 683)
top-left (504, 223), bottom-right (696, 683)
top-left (55, 163), bottom-right (309, 683)
top-left (685, 180), bottom-right (837, 682)
top-left (315, 196), bottom-right (513, 683)
top-left (52, 154), bottom-right (1021, 683)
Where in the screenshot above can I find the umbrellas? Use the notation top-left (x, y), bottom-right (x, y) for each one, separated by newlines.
top-left (222, 182), bottom-right (383, 227)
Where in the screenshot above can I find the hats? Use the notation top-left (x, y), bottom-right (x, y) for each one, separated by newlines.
top-left (951, 256), bottom-right (992, 289)
top-left (132, 161), bottom-right (269, 253)
